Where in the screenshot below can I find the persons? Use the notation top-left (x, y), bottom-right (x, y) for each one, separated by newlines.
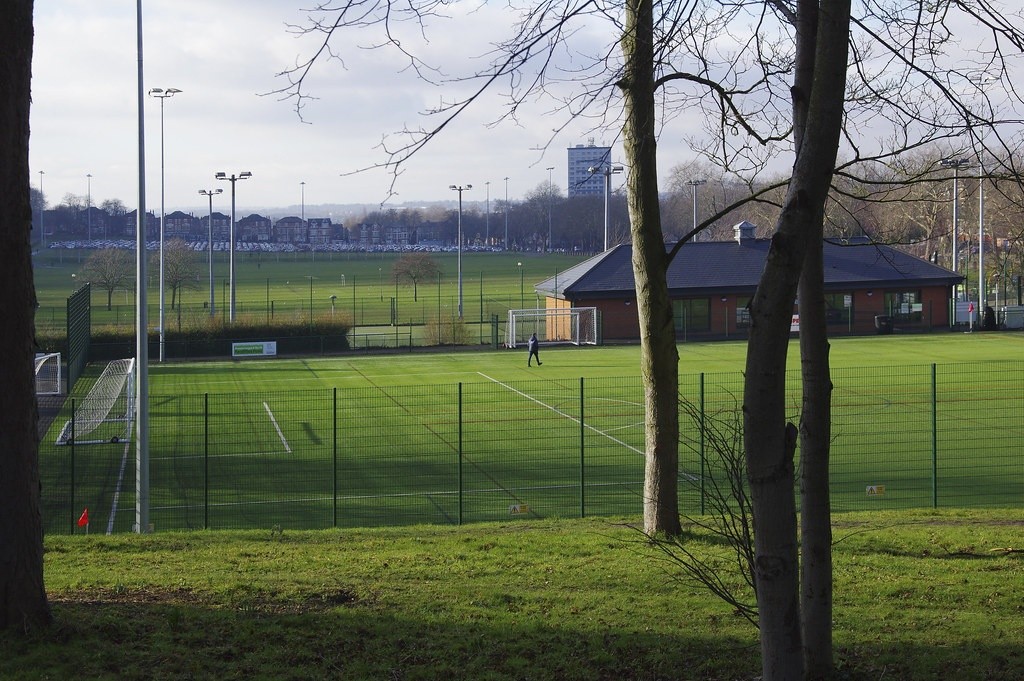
top-left (527, 333), bottom-right (543, 367)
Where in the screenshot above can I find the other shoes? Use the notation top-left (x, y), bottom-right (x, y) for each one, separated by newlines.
top-left (538, 362), bottom-right (542, 366)
top-left (528, 365), bottom-right (531, 367)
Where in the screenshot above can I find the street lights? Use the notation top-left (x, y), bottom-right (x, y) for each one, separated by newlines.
top-left (198, 188), bottom-right (224, 320)
top-left (214, 170), bottom-right (256, 326)
top-left (687, 178), bottom-right (708, 244)
top-left (589, 165), bottom-right (623, 253)
top-left (451, 183), bottom-right (472, 318)
top-left (935, 158), bottom-right (969, 327)
top-left (149, 84), bottom-right (184, 365)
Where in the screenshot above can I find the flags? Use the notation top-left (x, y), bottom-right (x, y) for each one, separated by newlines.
top-left (969, 302), bottom-right (974, 312)
top-left (77, 509), bottom-right (88, 528)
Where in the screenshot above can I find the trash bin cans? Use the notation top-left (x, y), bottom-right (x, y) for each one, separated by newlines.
top-left (874, 314), bottom-right (892, 334)
